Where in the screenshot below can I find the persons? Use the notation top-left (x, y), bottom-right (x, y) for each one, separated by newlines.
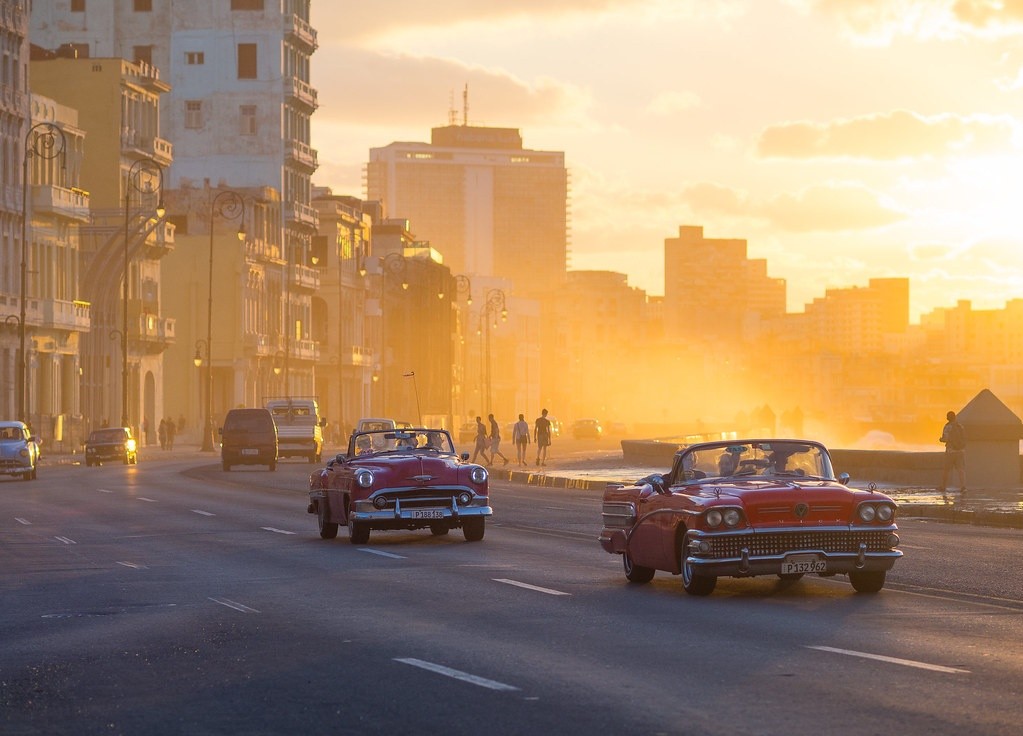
top-left (323, 421), bottom-right (352, 443)
top-left (534, 409), bottom-right (551, 465)
top-left (424, 433), bottom-right (444, 451)
top-left (145, 414), bottom-right (184, 451)
top-left (513, 414), bottom-right (530, 466)
top-left (358, 435), bottom-right (373, 452)
top-left (471, 414), bottom-right (508, 466)
top-left (938, 411), bottom-right (967, 493)
top-left (761, 453), bottom-right (804, 475)
top-left (669, 445), bottom-right (706, 480)
top-left (397, 438), bottom-right (417, 447)
top-left (719, 454), bottom-right (735, 475)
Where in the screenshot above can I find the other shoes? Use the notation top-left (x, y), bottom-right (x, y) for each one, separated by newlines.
top-left (522, 461), bottom-right (527, 466)
top-left (503, 459), bottom-right (509, 466)
top-left (536, 461), bottom-right (539, 466)
top-left (519, 464), bottom-right (521, 467)
top-left (485, 463), bottom-right (493, 466)
top-left (542, 463), bottom-right (546, 466)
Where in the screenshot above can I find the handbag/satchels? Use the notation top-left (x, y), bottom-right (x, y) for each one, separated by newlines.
top-left (520, 435), bottom-right (527, 444)
top-left (483, 432), bottom-right (492, 450)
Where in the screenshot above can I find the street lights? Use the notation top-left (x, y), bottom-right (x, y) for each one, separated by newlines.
top-left (371, 252), bottom-right (412, 418)
top-left (194, 189), bottom-right (246, 454)
top-left (18, 123), bottom-right (68, 421)
top-left (414, 265), bottom-right (510, 441)
top-left (121, 156), bottom-right (166, 426)
top-left (273, 220), bottom-right (321, 398)
top-left (328, 234), bottom-right (370, 445)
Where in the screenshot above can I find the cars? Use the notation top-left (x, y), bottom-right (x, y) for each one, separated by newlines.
top-left (306, 428), bottom-right (494, 545)
top-left (598, 439), bottom-right (904, 595)
top-left (84, 428), bottom-right (138, 466)
top-left (458, 416), bottom-right (629, 444)
top-left (0, 420), bottom-right (40, 481)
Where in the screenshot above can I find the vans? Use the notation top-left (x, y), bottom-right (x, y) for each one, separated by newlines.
top-left (218, 408), bottom-right (278, 471)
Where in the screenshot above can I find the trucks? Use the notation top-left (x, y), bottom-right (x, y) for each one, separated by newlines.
top-left (262, 394), bottom-right (331, 464)
